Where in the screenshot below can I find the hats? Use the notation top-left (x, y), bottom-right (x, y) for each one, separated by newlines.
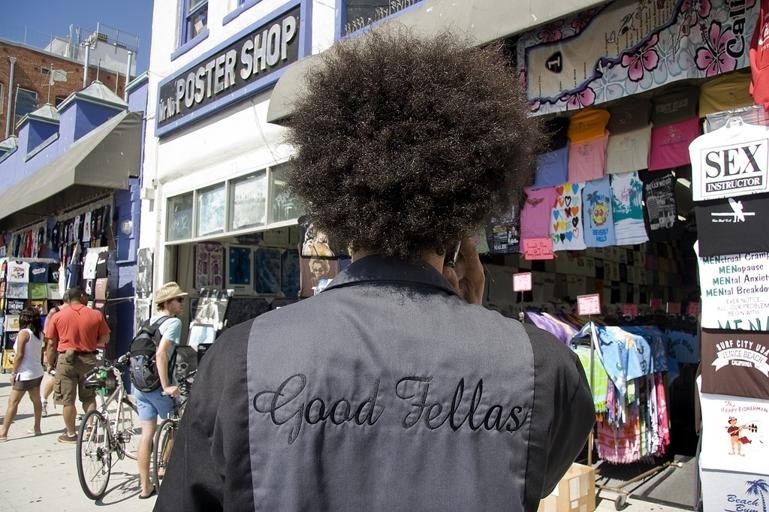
top-left (154, 281), bottom-right (188, 306)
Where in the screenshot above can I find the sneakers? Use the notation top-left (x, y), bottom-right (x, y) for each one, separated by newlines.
top-left (26, 428), bottom-right (42, 436)
top-left (57, 432), bottom-right (78, 444)
top-left (86, 426), bottom-right (98, 442)
top-left (41, 406), bottom-right (48, 417)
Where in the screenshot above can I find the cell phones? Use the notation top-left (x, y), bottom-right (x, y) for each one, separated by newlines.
top-left (444, 237), bottom-right (462, 267)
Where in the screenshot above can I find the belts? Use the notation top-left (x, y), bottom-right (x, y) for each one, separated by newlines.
top-left (58, 350), bottom-right (98, 356)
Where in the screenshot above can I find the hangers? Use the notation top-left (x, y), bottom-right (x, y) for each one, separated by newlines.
top-left (521, 302), bottom-right (676, 337)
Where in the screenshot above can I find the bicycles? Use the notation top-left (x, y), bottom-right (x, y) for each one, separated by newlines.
top-left (74, 350), bottom-right (141, 501)
top-left (152, 369), bottom-right (197, 496)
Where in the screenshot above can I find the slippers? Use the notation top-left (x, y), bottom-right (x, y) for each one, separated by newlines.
top-left (139, 485), bottom-right (155, 499)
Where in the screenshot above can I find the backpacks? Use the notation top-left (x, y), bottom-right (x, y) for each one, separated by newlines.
top-left (130, 316), bottom-right (178, 393)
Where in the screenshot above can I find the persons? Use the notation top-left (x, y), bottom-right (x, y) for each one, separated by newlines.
top-left (175, 346), bottom-right (197, 378)
top-left (40, 288), bottom-right (70, 418)
top-left (45, 287), bottom-right (110, 446)
top-left (0, 306), bottom-right (45, 441)
top-left (152, 27), bottom-right (595, 512)
top-left (126, 282), bottom-right (182, 500)
